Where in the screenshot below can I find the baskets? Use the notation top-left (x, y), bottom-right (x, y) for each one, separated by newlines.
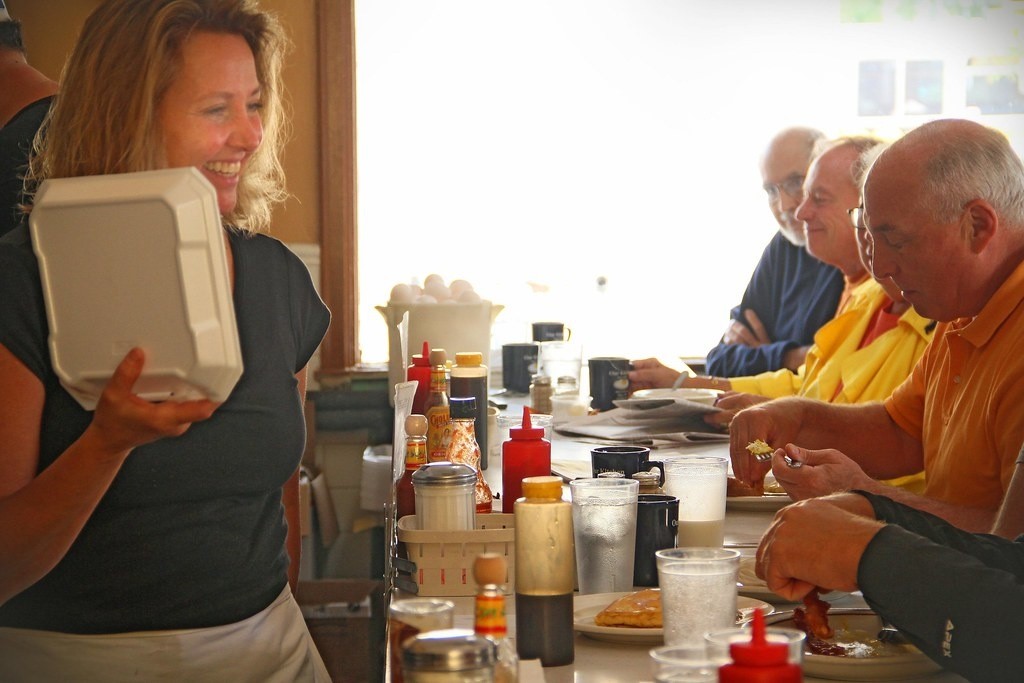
top-left (397, 511), bottom-right (515, 596)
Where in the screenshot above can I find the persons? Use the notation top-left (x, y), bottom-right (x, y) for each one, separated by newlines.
top-left (0, 0), bottom-right (336, 683)
top-left (622, 131), bottom-right (937, 436)
top-left (729, 122), bottom-right (1024, 683)
top-left (701, 124), bottom-right (842, 379)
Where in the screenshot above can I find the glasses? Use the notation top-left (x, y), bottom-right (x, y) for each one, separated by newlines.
top-left (845, 207), bottom-right (867, 230)
top-left (761, 176), bottom-right (806, 204)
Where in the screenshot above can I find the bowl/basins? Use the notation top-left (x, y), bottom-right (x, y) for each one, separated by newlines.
top-left (548, 394), bottom-right (593, 419)
top-left (633, 387), bottom-right (726, 407)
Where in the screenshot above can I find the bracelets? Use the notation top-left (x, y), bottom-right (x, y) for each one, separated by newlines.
top-left (672, 370), bottom-right (688, 391)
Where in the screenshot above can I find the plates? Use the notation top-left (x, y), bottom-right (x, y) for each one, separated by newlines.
top-left (567, 587), bottom-right (775, 644)
top-left (740, 604), bottom-right (945, 681)
top-left (725, 476), bottom-right (792, 511)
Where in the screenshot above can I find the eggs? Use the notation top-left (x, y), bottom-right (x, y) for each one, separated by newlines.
top-left (390, 273), bottom-right (483, 304)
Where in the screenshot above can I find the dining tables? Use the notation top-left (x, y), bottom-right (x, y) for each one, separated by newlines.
top-left (452, 364), bottom-right (968, 683)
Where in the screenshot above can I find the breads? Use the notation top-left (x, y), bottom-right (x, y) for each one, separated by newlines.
top-left (726, 477), bottom-right (755, 497)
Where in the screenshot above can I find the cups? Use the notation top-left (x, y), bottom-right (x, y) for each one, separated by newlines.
top-left (532, 322), bottom-right (571, 344)
top-left (588, 356), bottom-right (632, 412)
top-left (570, 445), bottom-right (807, 683)
top-left (383, 299), bottom-right (494, 407)
top-left (388, 597), bottom-right (455, 683)
top-left (536, 341), bottom-right (583, 391)
top-left (501, 343), bottom-right (539, 394)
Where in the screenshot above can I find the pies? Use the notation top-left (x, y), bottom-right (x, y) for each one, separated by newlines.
top-left (594, 588), bottom-right (664, 627)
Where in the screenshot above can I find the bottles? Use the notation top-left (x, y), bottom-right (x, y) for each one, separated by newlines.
top-left (717, 606), bottom-right (805, 683)
top-left (501, 405), bottom-right (552, 514)
top-left (514, 475), bottom-right (576, 668)
top-left (386, 551), bottom-right (520, 683)
top-left (528, 375), bottom-right (579, 415)
top-left (394, 341), bottom-right (488, 532)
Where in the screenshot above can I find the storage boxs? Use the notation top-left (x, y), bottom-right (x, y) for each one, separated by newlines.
top-left (298, 580), bottom-right (386, 683)
top-left (396, 513), bottom-right (516, 596)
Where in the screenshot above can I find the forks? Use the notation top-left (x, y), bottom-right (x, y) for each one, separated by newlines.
top-left (753, 452), bottom-right (804, 469)
